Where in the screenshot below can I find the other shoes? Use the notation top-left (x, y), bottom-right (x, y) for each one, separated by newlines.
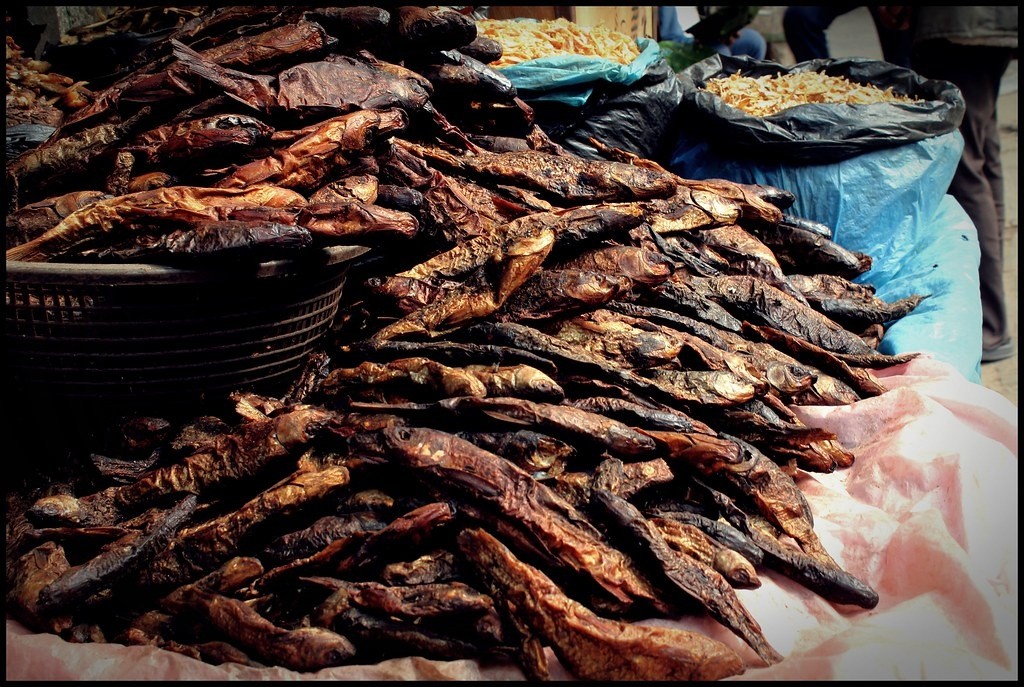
top-left (982, 337), bottom-right (1012, 362)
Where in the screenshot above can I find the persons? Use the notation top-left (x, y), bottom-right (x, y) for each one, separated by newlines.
top-left (659, 0), bottom-right (1019, 362)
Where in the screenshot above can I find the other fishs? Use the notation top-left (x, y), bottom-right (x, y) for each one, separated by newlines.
top-left (1, 1), bottom-right (933, 681)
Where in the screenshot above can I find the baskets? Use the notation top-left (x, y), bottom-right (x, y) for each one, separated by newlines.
top-left (6, 251), bottom-right (348, 421)
